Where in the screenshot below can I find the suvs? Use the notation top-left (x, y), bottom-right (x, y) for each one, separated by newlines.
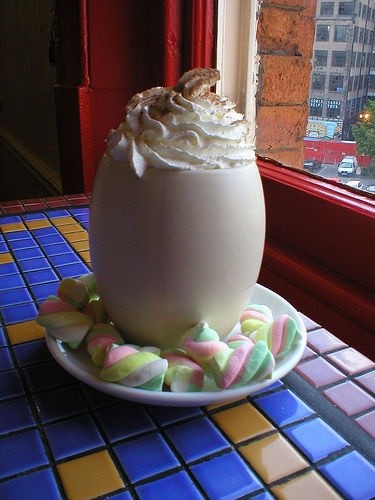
top-left (346, 181), bottom-right (364, 190)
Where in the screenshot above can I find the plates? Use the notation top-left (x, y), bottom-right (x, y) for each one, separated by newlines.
top-left (45, 272), bottom-right (308, 406)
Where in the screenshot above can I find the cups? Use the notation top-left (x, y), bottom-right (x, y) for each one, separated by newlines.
top-left (88, 154), bottom-right (266, 347)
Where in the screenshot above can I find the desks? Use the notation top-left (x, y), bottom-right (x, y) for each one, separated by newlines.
top-left (0, 195), bottom-right (375, 500)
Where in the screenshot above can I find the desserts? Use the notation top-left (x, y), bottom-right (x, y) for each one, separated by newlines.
top-left (106, 67), bottom-right (255, 178)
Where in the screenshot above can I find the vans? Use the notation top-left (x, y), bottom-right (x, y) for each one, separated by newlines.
top-left (338, 156), bottom-right (358, 178)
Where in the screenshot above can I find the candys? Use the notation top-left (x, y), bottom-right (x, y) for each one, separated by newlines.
top-left (37, 272), bottom-right (300, 392)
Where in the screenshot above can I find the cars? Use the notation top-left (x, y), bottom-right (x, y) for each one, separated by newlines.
top-left (356, 166), bottom-right (362, 176)
top-left (304, 160), bottom-right (321, 171)
top-left (366, 184), bottom-right (374, 192)
top-left (327, 177), bottom-right (340, 184)
